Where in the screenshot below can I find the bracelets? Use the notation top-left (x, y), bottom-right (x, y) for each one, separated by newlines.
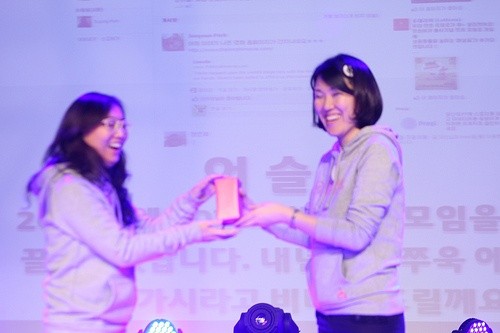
top-left (289, 209), bottom-right (299, 229)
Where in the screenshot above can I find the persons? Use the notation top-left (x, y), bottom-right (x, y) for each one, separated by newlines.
top-left (29, 91), bottom-right (236, 333)
top-left (234, 53), bottom-right (407, 333)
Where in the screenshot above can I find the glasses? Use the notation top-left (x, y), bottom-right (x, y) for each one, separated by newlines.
top-left (101, 117), bottom-right (127, 130)
